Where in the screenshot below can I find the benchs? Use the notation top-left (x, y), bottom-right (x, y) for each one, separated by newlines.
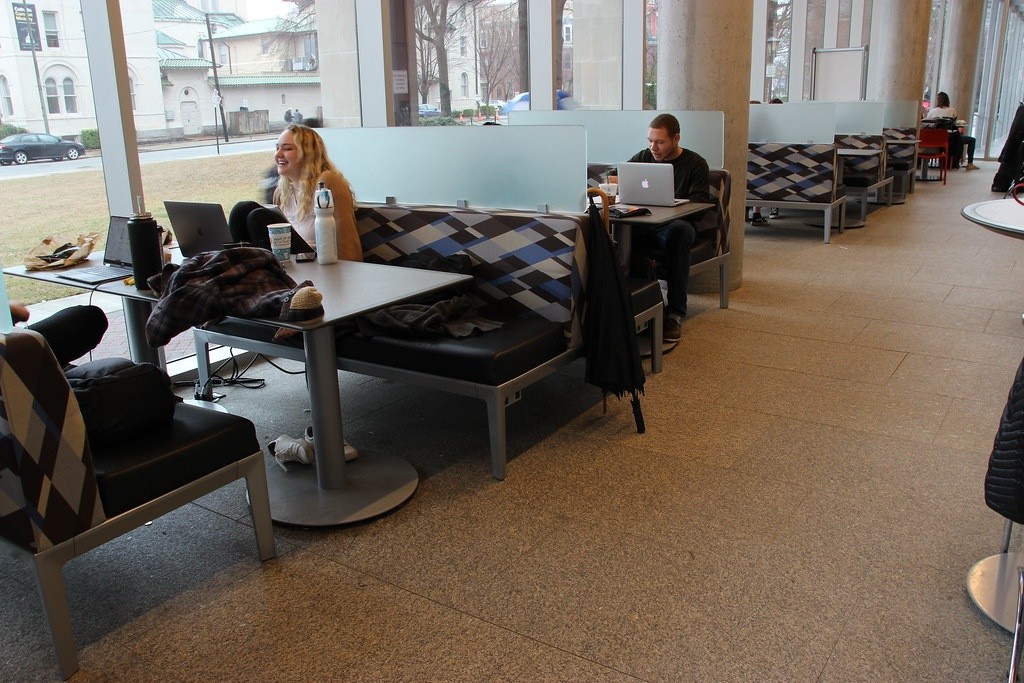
top-left (0, 123), bottom-right (921, 683)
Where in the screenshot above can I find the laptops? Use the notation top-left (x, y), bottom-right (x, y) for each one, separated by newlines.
top-left (56, 216), bottom-right (134, 285)
top-left (164, 201), bottom-right (234, 258)
top-left (617, 162), bottom-right (690, 207)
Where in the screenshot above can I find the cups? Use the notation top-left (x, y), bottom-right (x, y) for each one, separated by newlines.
top-left (267, 223), bottom-right (293, 262)
top-left (157, 225), bottom-right (165, 266)
top-left (599, 183), bottom-right (618, 207)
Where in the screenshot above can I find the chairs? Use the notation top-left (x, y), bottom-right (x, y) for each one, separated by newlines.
top-left (915, 129), bottom-right (948, 184)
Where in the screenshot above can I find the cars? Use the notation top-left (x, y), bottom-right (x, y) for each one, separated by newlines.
top-left (501, 92), bottom-right (529, 115)
top-left (482, 100), bottom-right (504, 112)
top-left (418, 104), bottom-right (440, 117)
top-left (0, 132), bottom-right (86, 166)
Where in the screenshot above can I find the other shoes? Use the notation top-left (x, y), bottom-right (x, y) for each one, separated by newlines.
top-left (752, 217), bottom-right (768, 226)
top-left (663, 317), bottom-right (682, 341)
top-left (769, 207), bottom-right (779, 219)
top-left (745, 210), bottom-right (749, 221)
top-left (966, 164), bottom-right (980, 170)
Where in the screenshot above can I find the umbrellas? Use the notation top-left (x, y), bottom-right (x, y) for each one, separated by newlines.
top-left (585, 188), bottom-right (646, 435)
top-left (501, 89), bottom-right (573, 114)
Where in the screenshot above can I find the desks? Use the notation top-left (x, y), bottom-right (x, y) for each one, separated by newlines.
top-left (866, 139), bottom-right (921, 205)
top-left (590, 195), bottom-right (715, 288)
top-left (802, 150), bottom-right (885, 228)
top-left (3, 249), bottom-right (474, 528)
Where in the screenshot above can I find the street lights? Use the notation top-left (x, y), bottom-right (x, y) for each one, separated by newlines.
top-left (766, 35), bottom-right (780, 102)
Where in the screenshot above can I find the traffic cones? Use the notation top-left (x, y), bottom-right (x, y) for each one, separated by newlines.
top-left (478, 112), bottom-right (482, 120)
top-left (494, 112), bottom-right (499, 120)
top-left (459, 113), bottom-right (463, 121)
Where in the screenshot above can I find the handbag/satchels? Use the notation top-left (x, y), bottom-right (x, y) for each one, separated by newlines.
top-left (61, 357), bottom-right (182, 451)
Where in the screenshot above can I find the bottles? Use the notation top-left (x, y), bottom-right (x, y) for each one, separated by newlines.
top-left (313, 181), bottom-right (339, 264)
top-left (127, 196), bottom-right (162, 289)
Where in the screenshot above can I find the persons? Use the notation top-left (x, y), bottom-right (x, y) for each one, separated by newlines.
top-left (191, 123), bottom-right (363, 340)
top-left (10, 302), bottom-right (108, 370)
top-left (744, 98), bottom-right (783, 227)
top-left (284, 108), bottom-right (301, 124)
top-left (608, 114), bottom-right (710, 342)
top-left (926, 92), bottom-right (979, 170)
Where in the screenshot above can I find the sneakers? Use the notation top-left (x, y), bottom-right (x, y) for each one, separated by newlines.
top-left (267, 433), bottom-right (314, 472)
top-left (304, 426), bottom-right (358, 461)
